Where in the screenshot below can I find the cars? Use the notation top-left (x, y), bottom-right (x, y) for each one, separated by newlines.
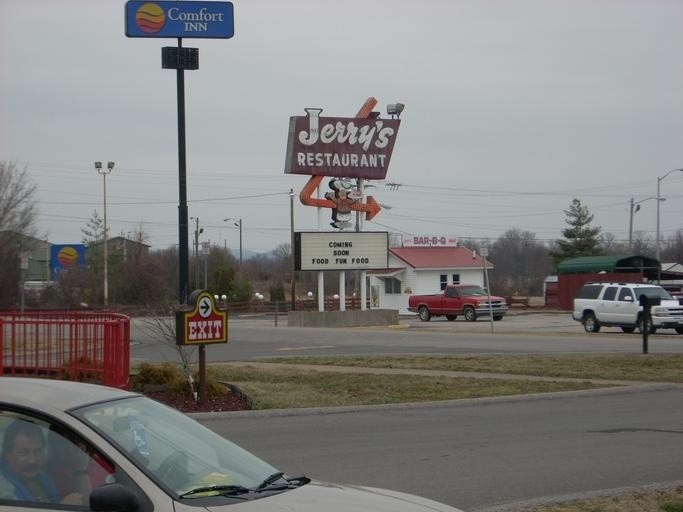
top-left (0, 375), bottom-right (462, 512)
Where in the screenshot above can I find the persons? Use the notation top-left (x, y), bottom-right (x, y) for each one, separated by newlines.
top-left (39, 421), bottom-right (118, 501)
top-left (0, 416), bottom-right (85, 510)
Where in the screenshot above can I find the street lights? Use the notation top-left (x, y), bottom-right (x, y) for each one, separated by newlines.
top-left (95, 160), bottom-right (115, 308)
top-left (655, 167), bottom-right (682, 262)
top-left (188, 216), bottom-right (203, 289)
top-left (223, 217), bottom-right (243, 283)
top-left (627, 195), bottom-right (666, 254)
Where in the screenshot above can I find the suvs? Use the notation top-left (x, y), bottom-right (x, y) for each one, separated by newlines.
top-left (572, 280), bottom-right (682, 335)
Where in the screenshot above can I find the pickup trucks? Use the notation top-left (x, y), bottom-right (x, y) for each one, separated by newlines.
top-left (406, 284), bottom-right (508, 321)
